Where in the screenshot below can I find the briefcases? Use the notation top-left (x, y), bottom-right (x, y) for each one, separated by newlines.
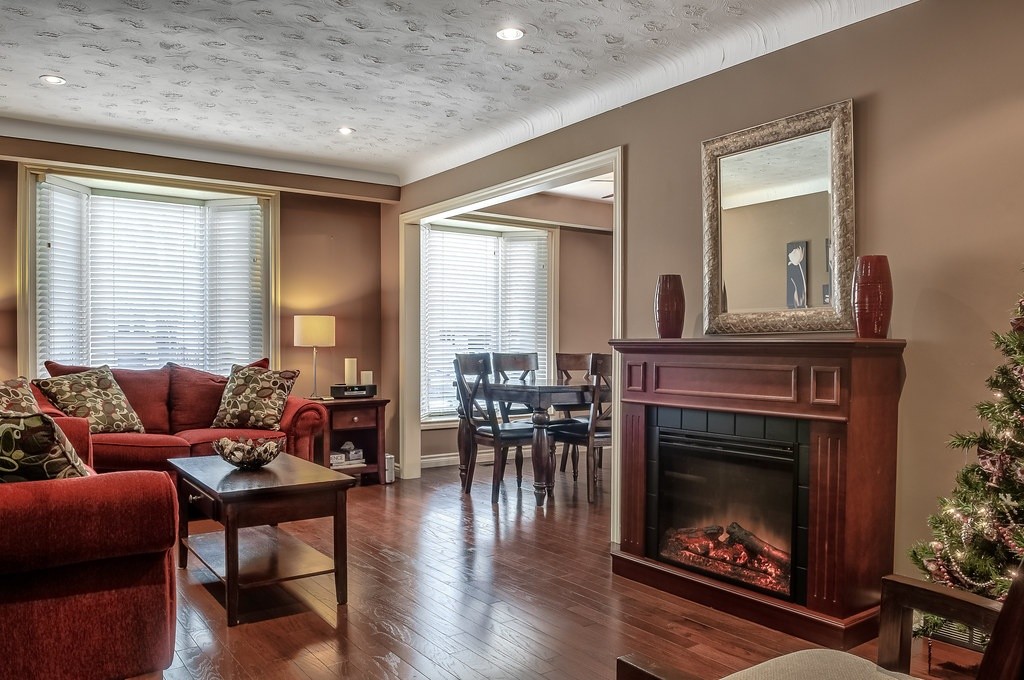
top-left (385, 453), bottom-right (395, 483)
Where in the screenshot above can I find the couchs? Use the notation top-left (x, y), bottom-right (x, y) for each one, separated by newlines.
top-left (0, 357), bottom-right (328, 679)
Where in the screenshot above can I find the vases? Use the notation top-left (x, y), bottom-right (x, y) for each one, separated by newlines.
top-left (851, 255), bottom-right (894, 338)
top-left (654, 275), bottom-right (685, 338)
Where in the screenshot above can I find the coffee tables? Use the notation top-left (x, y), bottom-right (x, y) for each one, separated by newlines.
top-left (167, 449), bottom-right (357, 627)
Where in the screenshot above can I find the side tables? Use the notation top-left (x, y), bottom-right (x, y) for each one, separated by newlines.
top-left (302, 395), bottom-right (390, 485)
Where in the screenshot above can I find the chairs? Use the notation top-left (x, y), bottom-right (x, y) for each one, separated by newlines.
top-left (615, 553), bottom-right (1024, 680)
top-left (452, 353), bottom-right (612, 506)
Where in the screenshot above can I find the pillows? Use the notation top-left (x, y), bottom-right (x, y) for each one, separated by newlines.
top-left (0, 375), bottom-right (40, 418)
top-left (32, 363), bottom-right (146, 437)
top-left (210, 363), bottom-right (300, 432)
top-left (0, 412), bottom-right (90, 484)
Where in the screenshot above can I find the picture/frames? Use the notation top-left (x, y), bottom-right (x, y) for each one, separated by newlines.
top-left (787, 241), bottom-right (808, 308)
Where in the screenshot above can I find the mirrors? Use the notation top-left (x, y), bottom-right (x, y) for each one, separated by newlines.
top-left (700, 98), bottom-right (857, 335)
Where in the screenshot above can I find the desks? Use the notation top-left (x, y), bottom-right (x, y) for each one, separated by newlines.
top-left (453, 381), bottom-right (612, 506)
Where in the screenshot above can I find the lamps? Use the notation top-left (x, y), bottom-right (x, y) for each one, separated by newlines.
top-left (293, 315), bottom-right (336, 401)
